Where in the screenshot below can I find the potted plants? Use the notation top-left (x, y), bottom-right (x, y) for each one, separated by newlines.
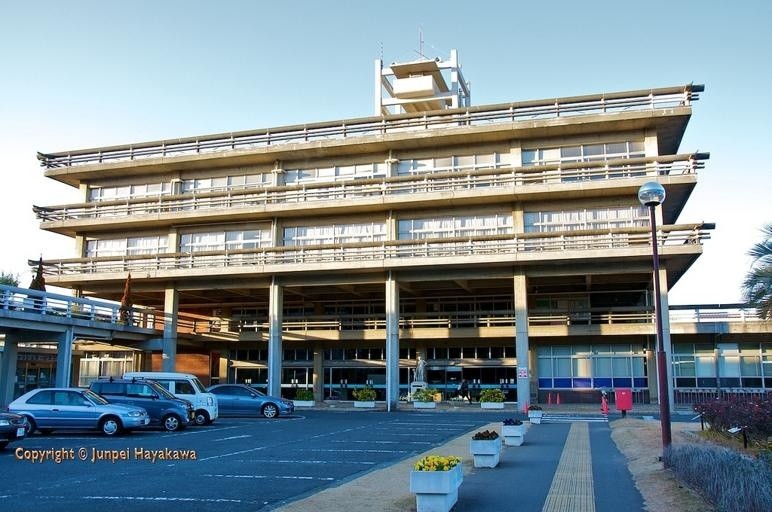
top-left (480, 390), bottom-right (505, 410)
top-left (352, 390), bottom-right (377, 408)
top-left (411, 389), bottom-right (436, 409)
top-left (293, 389), bottom-right (316, 407)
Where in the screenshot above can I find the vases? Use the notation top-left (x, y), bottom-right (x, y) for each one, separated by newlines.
top-left (527, 411), bottom-right (542, 424)
top-left (410, 462), bottom-right (464, 512)
top-left (471, 437), bottom-right (500, 469)
top-left (503, 426), bottom-right (524, 446)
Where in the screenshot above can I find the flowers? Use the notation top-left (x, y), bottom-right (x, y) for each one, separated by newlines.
top-left (503, 418), bottom-right (522, 425)
top-left (471, 430), bottom-right (498, 440)
top-left (528, 404), bottom-right (542, 410)
top-left (413, 456), bottom-right (462, 472)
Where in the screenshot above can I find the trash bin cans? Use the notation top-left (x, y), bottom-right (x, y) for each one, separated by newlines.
top-left (615, 388), bottom-right (633, 417)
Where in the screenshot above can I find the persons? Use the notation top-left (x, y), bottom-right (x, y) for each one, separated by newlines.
top-left (471, 384), bottom-right (482, 402)
top-left (458, 378), bottom-right (472, 404)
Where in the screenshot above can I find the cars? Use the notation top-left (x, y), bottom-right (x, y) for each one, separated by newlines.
top-left (0, 372), bottom-right (295, 455)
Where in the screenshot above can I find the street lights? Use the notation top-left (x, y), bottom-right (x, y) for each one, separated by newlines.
top-left (638, 180), bottom-right (672, 469)
